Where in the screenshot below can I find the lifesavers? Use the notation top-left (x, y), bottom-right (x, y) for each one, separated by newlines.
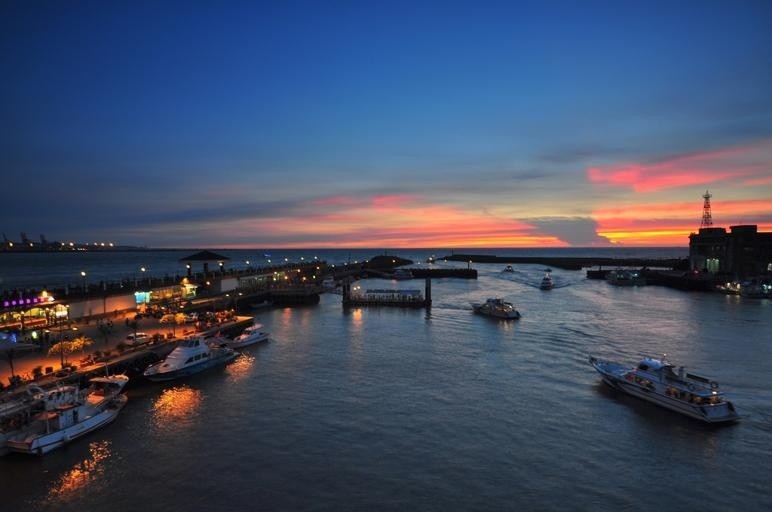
top-left (711, 381), bottom-right (718, 389)
top-left (688, 383), bottom-right (696, 391)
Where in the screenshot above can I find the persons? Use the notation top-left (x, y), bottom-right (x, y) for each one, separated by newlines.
top-left (72, 409), bottom-right (79, 424)
top-left (664, 386), bottom-right (678, 398)
top-left (687, 394), bottom-right (694, 403)
top-left (645, 378), bottom-right (654, 388)
top-left (133, 304), bottom-right (163, 321)
top-left (38, 331), bottom-right (74, 349)
top-left (94, 346), bottom-right (111, 362)
top-left (25, 337), bottom-right (34, 343)
top-left (114, 309), bottom-right (129, 327)
top-left (709, 397), bottom-right (723, 404)
top-left (96, 316), bottom-right (114, 329)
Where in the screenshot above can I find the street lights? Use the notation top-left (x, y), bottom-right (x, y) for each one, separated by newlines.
top-left (56, 310), bottom-right (67, 365)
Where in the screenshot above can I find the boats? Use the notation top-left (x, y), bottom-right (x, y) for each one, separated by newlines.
top-left (607, 271), bottom-right (647, 285)
top-left (541, 278), bottom-right (553, 289)
top-left (472, 299), bottom-right (521, 320)
top-left (716, 281), bottom-right (772, 298)
top-left (504, 266), bottom-right (513, 273)
top-left (589, 357), bottom-right (742, 423)
top-left (394, 270), bottom-right (414, 279)
top-left (5, 323), bottom-right (270, 458)
